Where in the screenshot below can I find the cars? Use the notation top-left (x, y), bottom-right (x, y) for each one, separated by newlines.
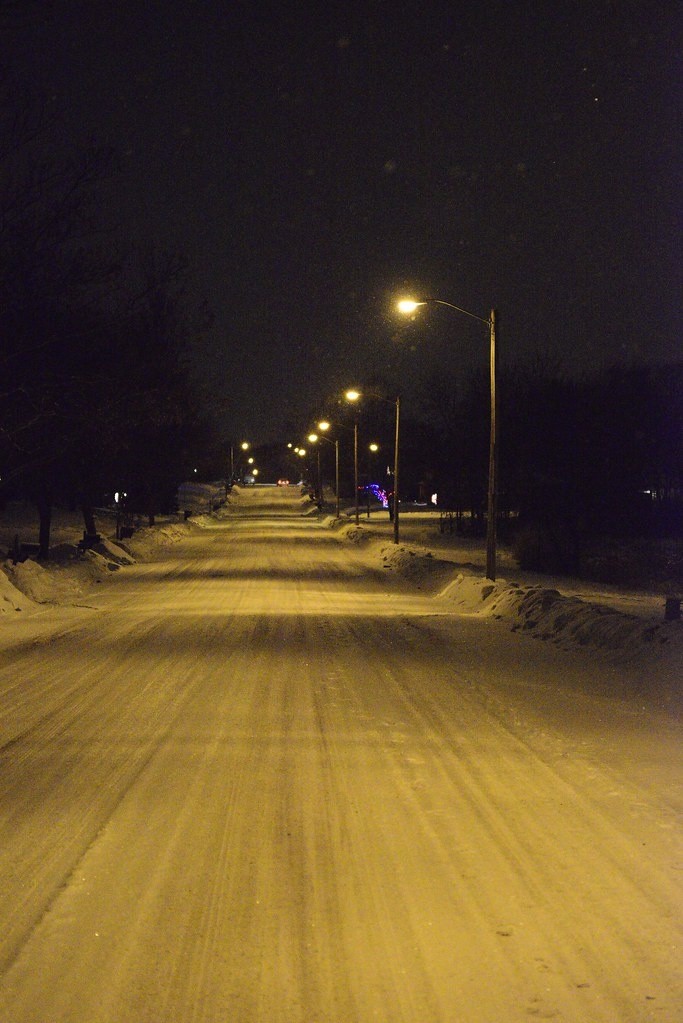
top-left (244, 476), bottom-right (255, 486)
top-left (278, 478), bottom-right (289, 488)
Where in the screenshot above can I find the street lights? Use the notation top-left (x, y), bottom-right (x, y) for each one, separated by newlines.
top-left (346, 389), bottom-right (401, 544)
top-left (231, 442), bottom-right (249, 487)
top-left (247, 458), bottom-right (258, 476)
top-left (287, 420), bottom-right (346, 530)
top-left (400, 298), bottom-right (498, 583)
top-left (309, 434), bottom-right (340, 518)
